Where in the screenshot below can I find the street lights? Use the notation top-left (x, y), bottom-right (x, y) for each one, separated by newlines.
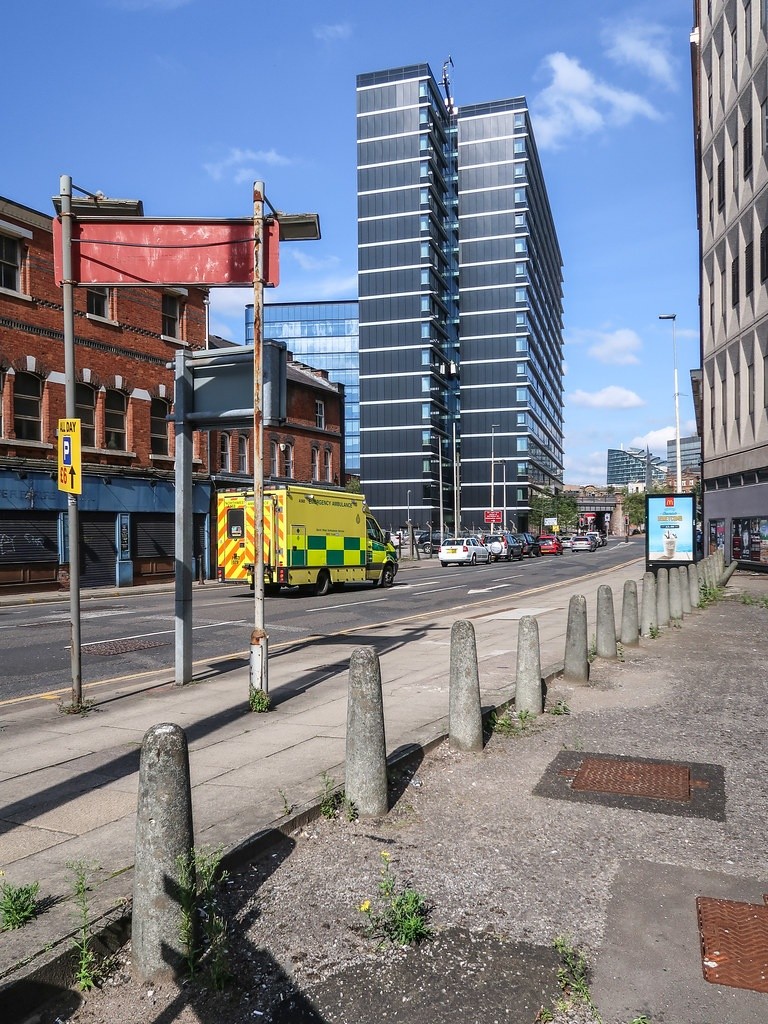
top-left (435, 435), bottom-right (443, 543)
top-left (490, 424), bottom-right (499, 534)
top-left (659, 314), bottom-right (684, 492)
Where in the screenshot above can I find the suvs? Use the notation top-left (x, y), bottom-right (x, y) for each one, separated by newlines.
top-left (512, 532), bottom-right (541, 557)
top-left (481, 535), bottom-right (523, 561)
top-left (418, 531), bottom-right (456, 555)
top-left (437, 537), bottom-right (493, 565)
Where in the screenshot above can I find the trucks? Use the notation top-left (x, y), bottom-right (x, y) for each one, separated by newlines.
top-left (214, 484), bottom-right (399, 595)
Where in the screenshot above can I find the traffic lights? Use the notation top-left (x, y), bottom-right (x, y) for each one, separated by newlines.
top-left (580, 518), bottom-right (584, 527)
top-left (625, 516), bottom-right (629, 525)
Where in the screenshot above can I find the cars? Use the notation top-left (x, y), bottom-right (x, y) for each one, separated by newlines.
top-left (537, 535), bottom-right (564, 556)
top-left (587, 532), bottom-right (602, 546)
top-left (403, 530), bottom-right (426, 544)
top-left (572, 536), bottom-right (596, 552)
top-left (367, 528), bottom-right (400, 549)
top-left (561, 537), bottom-right (574, 548)
